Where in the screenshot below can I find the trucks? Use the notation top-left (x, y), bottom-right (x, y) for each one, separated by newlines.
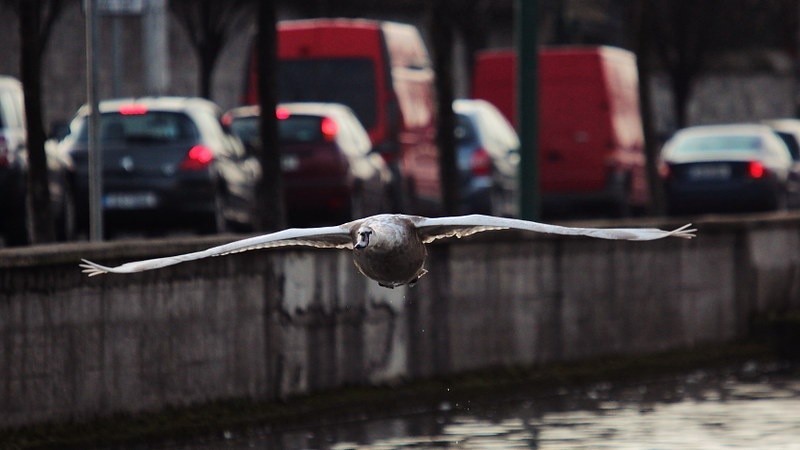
top-left (247, 17), bottom-right (442, 196)
top-left (468, 45), bottom-right (650, 209)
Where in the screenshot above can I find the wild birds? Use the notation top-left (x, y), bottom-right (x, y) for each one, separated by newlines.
top-left (80, 214), bottom-right (697, 290)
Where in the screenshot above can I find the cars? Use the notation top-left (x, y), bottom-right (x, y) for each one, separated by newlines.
top-left (449, 98), bottom-right (521, 217)
top-left (231, 102), bottom-right (396, 225)
top-left (0, 75), bottom-right (264, 237)
top-left (646, 116), bottom-right (800, 217)
